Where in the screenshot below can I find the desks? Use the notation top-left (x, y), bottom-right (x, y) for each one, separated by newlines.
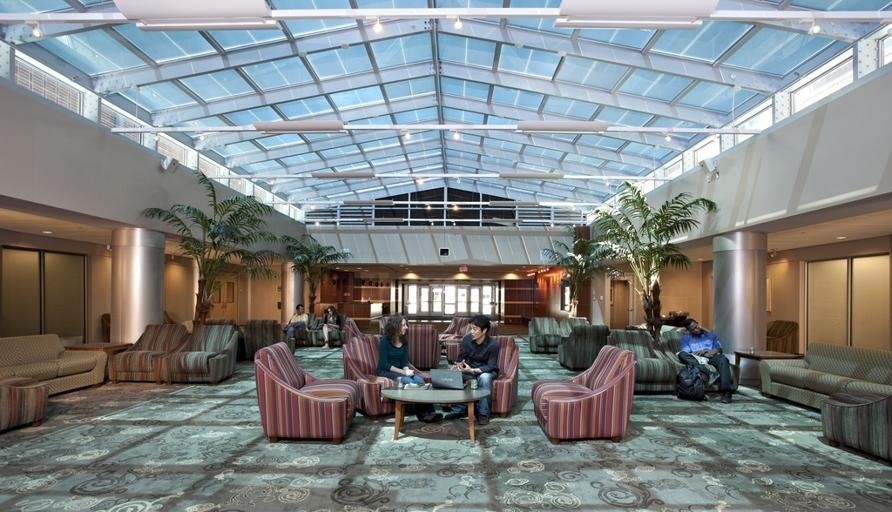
top-left (732, 349), bottom-right (805, 384)
top-left (65, 342), bottom-right (133, 353)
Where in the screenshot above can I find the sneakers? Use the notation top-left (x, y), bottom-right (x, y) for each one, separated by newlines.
top-left (445, 412), bottom-right (465, 420)
top-left (477, 413), bottom-right (489, 425)
top-left (708, 371), bottom-right (720, 386)
top-left (722, 391), bottom-right (731, 403)
top-left (424, 413), bottom-right (443, 423)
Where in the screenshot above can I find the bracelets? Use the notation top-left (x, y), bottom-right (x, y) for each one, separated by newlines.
top-left (472, 367), bottom-right (475, 375)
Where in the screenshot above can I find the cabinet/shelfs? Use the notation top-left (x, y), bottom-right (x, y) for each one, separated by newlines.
top-left (353, 285), bottom-right (398, 303)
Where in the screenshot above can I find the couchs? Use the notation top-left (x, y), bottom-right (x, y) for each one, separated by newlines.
top-left (0, 333), bottom-right (107, 396)
top-left (758, 341), bottom-right (892, 410)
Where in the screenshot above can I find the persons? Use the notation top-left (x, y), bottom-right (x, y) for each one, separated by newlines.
top-left (678, 318), bottom-right (733, 404)
top-left (283, 303), bottom-right (309, 345)
top-left (319, 306), bottom-right (341, 349)
top-left (445, 314), bottom-right (501, 425)
top-left (376, 313), bottom-right (443, 423)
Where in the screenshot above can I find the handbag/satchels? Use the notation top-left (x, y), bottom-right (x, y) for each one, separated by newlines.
top-left (676, 364), bottom-right (707, 401)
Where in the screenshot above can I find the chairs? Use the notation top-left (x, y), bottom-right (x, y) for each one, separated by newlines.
top-left (347, 312), bottom-right (497, 369)
top-left (0, 377), bottom-right (49, 430)
top-left (820, 390), bottom-right (892, 465)
top-left (440, 336), bottom-right (520, 418)
top-left (205, 311), bottom-right (362, 360)
top-left (342, 334), bottom-right (431, 420)
top-left (107, 324), bottom-right (189, 385)
top-left (767, 319), bottom-right (800, 355)
top-left (531, 345), bottom-right (638, 444)
top-left (160, 324), bottom-right (240, 385)
top-left (527, 315), bottom-right (741, 394)
top-left (254, 341), bottom-right (361, 444)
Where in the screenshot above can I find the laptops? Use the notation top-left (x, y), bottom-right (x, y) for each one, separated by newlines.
top-left (430, 368), bottom-right (467, 390)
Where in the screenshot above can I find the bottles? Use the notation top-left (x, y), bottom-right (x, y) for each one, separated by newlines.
top-left (470, 371), bottom-right (478, 390)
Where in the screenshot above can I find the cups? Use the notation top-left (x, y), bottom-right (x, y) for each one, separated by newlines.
top-left (749, 345), bottom-right (755, 355)
top-left (397, 377), bottom-right (404, 389)
